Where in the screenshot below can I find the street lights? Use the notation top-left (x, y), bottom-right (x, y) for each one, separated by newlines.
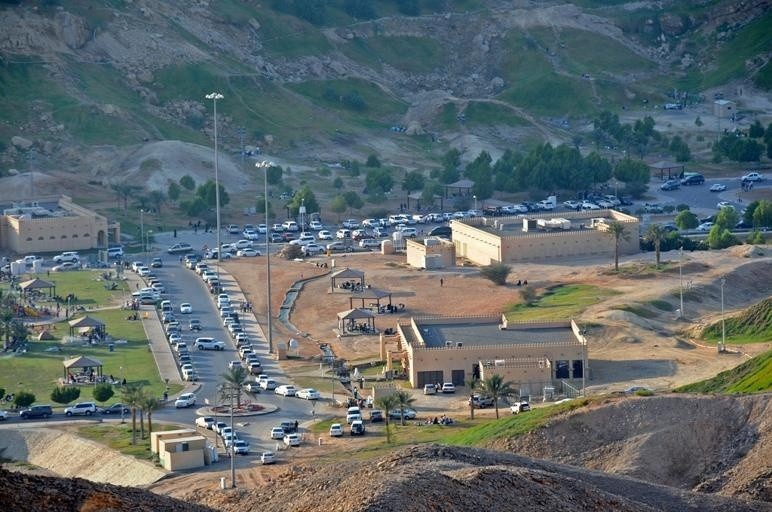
top-left (147, 230), bottom-right (153, 252)
top-left (255, 159), bottom-right (279, 352)
top-left (227, 382), bottom-right (244, 488)
top-left (203, 91), bottom-right (228, 289)
top-left (677, 246), bottom-right (685, 320)
top-left (720, 276), bottom-right (728, 350)
top-left (318, 353), bottom-right (336, 406)
top-left (140, 209), bottom-right (145, 252)
top-left (472, 195), bottom-right (477, 210)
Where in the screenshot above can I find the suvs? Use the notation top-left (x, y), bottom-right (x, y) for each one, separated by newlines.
top-left (19, 404), bottom-right (53, 419)
top-left (350, 420), bottom-right (365, 434)
top-left (64, 401), bottom-right (98, 417)
top-left (468, 393), bottom-right (494, 408)
top-left (510, 401), bottom-right (529, 414)
top-left (681, 175), bottom-right (704, 185)
top-left (195, 337), bottom-right (225, 351)
top-left (644, 202), bottom-right (663, 213)
top-left (0, 246), bottom-right (128, 276)
top-left (441, 382), bottom-right (456, 393)
top-left (174, 392), bottom-right (197, 409)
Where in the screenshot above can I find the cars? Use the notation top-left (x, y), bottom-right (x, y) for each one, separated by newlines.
top-left (128, 257), bottom-right (202, 381)
top-left (388, 408), bottom-right (416, 419)
top-left (706, 183), bottom-right (727, 192)
top-left (696, 201), bottom-right (772, 232)
top-left (242, 374), bottom-right (320, 401)
top-left (260, 451), bottom-right (276, 464)
top-left (644, 221), bottom-right (679, 234)
top-left (659, 180), bottom-right (682, 191)
top-left (218, 292), bottom-right (264, 374)
top-left (565, 183), bottom-right (632, 216)
top-left (196, 415), bottom-right (249, 455)
top-left (167, 242), bottom-right (222, 294)
top-left (271, 419), bottom-right (301, 446)
top-left (102, 402), bottom-right (129, 413)
top-left (740, 173), bottom-right (762, 182)
top-left (0, 408), bottom-right (10, 422)
top-left (369, 410), bottom-right (382, 422)
top-left (554, 385), bottom-right (658, 404)
top-left (330, 423), bottom-right (344, 437)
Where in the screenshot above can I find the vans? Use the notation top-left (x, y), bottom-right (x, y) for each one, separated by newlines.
top-left (424, 383), bottom-right (437, 395)
top-left (346, 406), bottom-right (362, 423)
